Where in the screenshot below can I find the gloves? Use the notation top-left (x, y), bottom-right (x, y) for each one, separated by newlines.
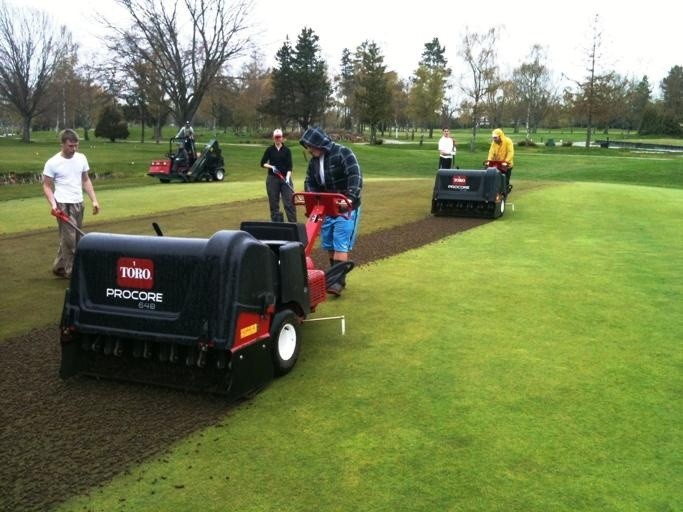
top-left (271, 165), bottom-right (289, 187)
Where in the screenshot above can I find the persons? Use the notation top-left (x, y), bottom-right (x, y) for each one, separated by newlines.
top-left (485, 128), bottom-right (513, 197)
top-left (298, 125), bottom-right (362, 296)
top-left (260, 128), bottom-right (296, 222)
top-left (175, 119), bottom-right (194, 153)
top-left (41, 129), bottom-right (100, 280)
top-left (437, 126), bottom-right (456, 168)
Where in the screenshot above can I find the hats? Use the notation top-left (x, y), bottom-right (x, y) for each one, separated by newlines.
top-left (273, 129), bottom-right (282, 138)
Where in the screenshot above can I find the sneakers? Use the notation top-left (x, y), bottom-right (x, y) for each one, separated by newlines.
top-left (327, 281), bottom-right (345, 295)
top-left (52, 266), bottom-right (73, 280)
